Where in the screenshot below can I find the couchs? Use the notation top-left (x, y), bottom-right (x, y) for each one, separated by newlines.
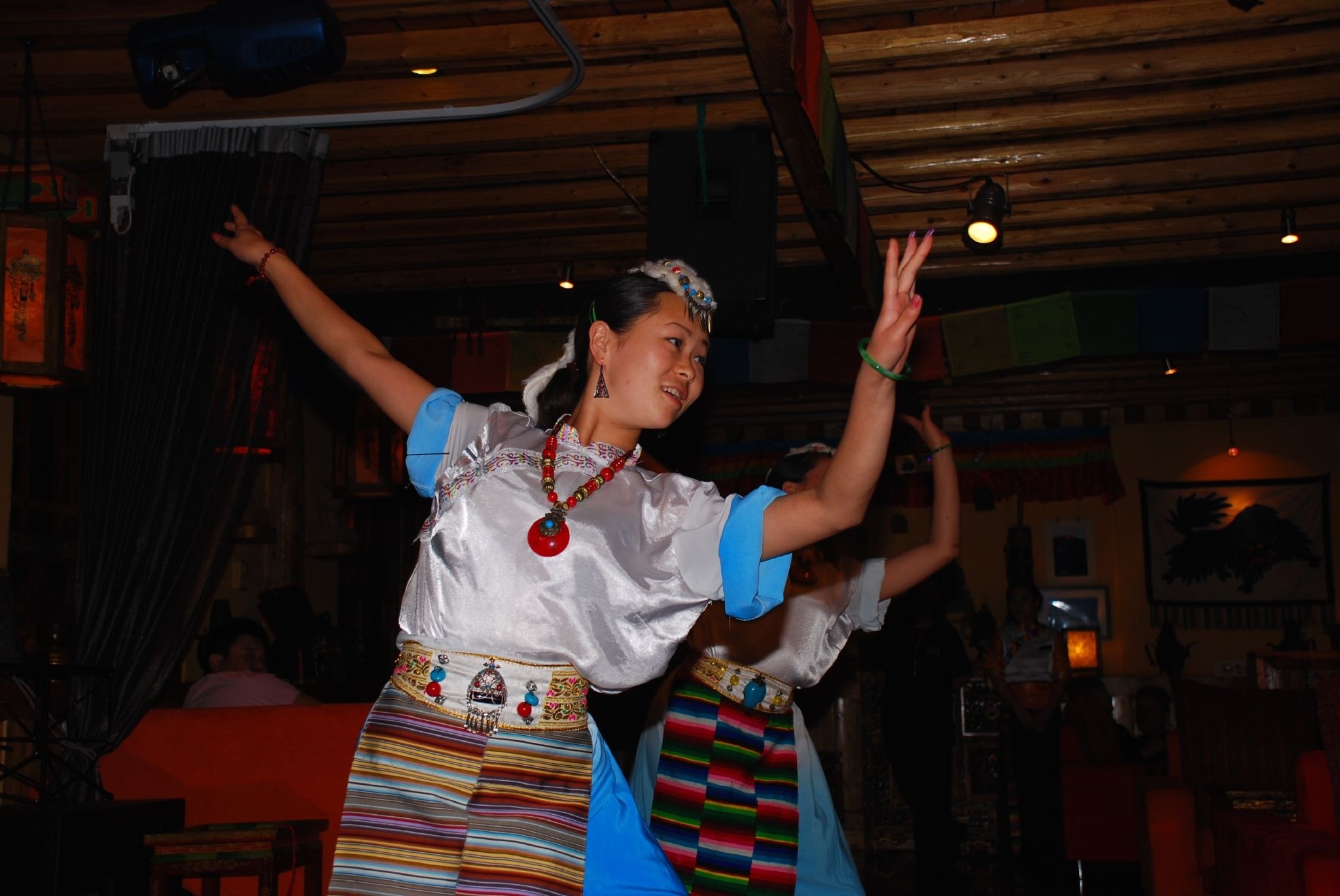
top-left (91, 702), bottom-right (384, 896)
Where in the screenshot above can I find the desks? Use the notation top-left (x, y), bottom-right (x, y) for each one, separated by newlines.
top-left (1198, 803), bottom-right (1303, 896)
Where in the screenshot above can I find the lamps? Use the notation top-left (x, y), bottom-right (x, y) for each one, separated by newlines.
top-left (964, 183), bottom-right (1006, 244)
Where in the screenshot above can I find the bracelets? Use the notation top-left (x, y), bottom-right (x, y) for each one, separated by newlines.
top-left (858, 337), bottom-right (912, 380)
top-left (931, 443), bottom-right (952, 456)
top-left (246, 247), bottom-right (287, 286)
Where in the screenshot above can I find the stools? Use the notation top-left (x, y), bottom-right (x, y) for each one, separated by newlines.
top-left (143, 820), bottom-right (327, 896)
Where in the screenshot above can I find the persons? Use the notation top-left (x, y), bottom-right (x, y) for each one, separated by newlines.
top-left (215, 205), bottom-right (935, 896)
top-left (883, 579), bottom-right (973, 869)
top-left (628, 405), bottom-right (961, 896)
top-left (181, 617), bottom-right (317, 708)
top-left (984, 579), bottom-right (1068, 864)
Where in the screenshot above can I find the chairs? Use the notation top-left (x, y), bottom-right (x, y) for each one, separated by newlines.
top-left (1060, 725), bottom-right (1340, 896)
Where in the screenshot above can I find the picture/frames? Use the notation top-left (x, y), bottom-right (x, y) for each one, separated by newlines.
top-left (1036, 587), bottom-right (1107, 636)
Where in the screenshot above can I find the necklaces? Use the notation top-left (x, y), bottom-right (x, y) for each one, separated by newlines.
top-left (528, 414), bottom-right (633, 557)
